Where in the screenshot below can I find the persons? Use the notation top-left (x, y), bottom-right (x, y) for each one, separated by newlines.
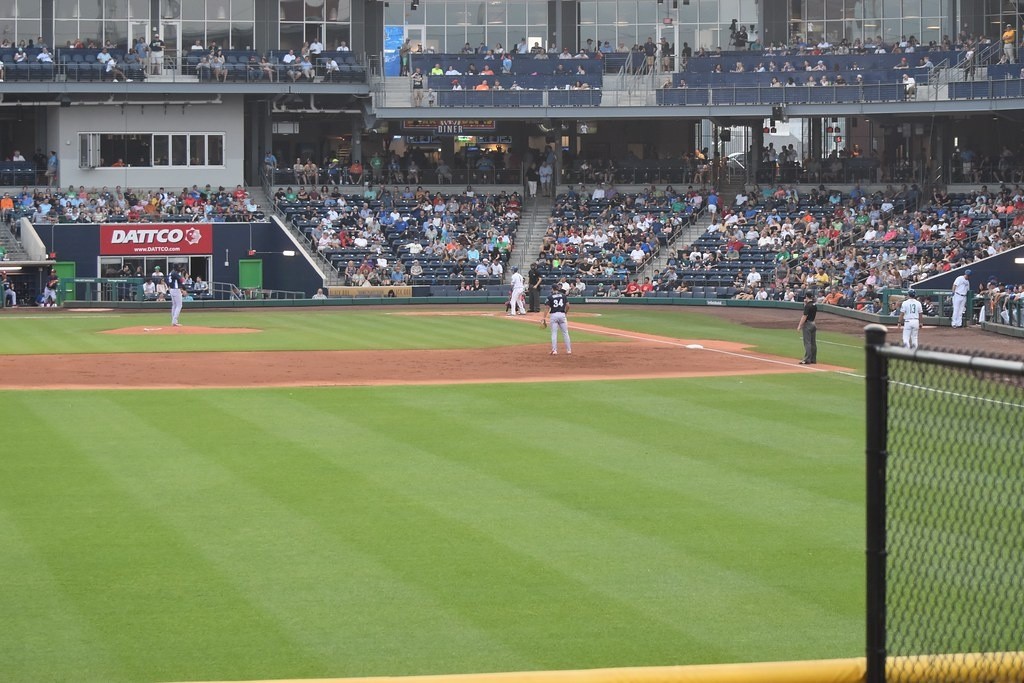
top-left (399, 23), bottom-right (1024, 107)
top-left (974, 276), bottom-right (1024, 327)
top-left (112, 158), bottom-right (126, 167)
top-left (283, 38), bottom-right (349, 84)
top-left (169, 263), bottom-right (186, 326)
top-left (192, 156), bottom-right (201, 165)
top-left (0, 36), bottom-right (55, 82)
top-left (897, 289), bottom-right (923, 349)
top-left (797, 292), bottom-right (817, 364)
top-left (951, 270), bottom-right (972, 328)
top-left (0, 185), bottom-right (266, 307)
top-left (247, 56), bottom-right (276, 83)
top-left (0, 148), bottom-right (57, 188)
top-left (191, 40), bottom-right (228, 83)
top-left (264, 143), bottom-right (1024, 316)
top-left (541, 284), bottom-right (571, 354)
top-left (66, 34), bottom-right (166, 83)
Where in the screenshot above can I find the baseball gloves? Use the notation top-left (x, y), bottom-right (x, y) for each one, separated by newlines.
top-left (538, 318), bottom-right (547, 329)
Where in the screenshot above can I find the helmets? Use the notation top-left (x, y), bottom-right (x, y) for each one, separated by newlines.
top-left (510, 266), bottom-right (518, 272)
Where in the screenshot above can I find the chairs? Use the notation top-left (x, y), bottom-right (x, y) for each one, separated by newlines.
top-left (271, 167), bottom-right (694, 184)
top-left (0, 190), bottom-right (1024, 324)
top-left (0, 161), bottom-right (47, 186)
top-left (186, 49), bottom-right (365, 84)
top-left (761, 158), bottom-right (1024, 184)
top-left (410, 43), bottom-right (1024, 107)
top-left (0, 47), bottom-right (147, 81)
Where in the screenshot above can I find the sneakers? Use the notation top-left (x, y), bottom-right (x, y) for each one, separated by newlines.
top-left (566, 351), bottom-right (571, 354)
top-left (549, 351), bottom-right (557, 355)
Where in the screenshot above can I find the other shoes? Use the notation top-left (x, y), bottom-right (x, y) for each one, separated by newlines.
top-left (799, 360), bottom-right (816, 364)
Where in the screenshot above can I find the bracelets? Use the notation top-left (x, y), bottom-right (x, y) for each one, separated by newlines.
top-left (898, 322), bottom-right (901, 323)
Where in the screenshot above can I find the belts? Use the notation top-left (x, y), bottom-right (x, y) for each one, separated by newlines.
top-left (956, 293), bottom-right (967, 296)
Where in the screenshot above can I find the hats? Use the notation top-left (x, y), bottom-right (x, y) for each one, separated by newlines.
top-left (1004, 285), bottom-right (1014, 289)
top-left (369, 210), bottom-right (389, 220)
top-left (552, 284), bottom-right (559, 290)
top-left (332, 159), bottom-right (339, 162)
top-left (609, 225), bottom-right (615, 228)
top-left (804, 291), bottom-right (813, 299)
top-left (869, 269), bottom-right (875, 272)
top-left (908, 289), bottom-right (916, 296)
top-left (385, 191), bottom-right (389, 193)
